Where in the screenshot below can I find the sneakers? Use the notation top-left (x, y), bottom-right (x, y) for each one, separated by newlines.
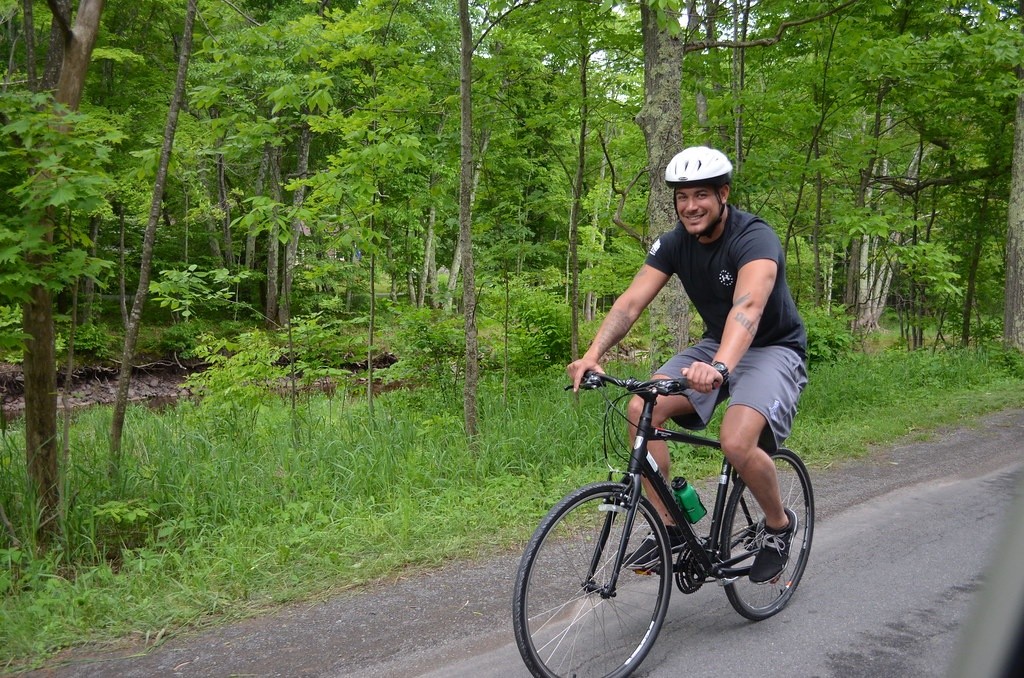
top-left (749, 508), bottom-right (799, 585)
top-left (621, 525), bottom-right (697, 570)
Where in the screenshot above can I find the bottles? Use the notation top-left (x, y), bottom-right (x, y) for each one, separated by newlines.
top-left (670, 476), bottom-right (707, 524)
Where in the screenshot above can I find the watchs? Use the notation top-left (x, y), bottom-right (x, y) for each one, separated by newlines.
top-left (708, 361), bottom-right (729, 388)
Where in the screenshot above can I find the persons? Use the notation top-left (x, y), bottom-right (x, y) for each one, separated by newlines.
top-left (567, 146), bottom-right (808, 584)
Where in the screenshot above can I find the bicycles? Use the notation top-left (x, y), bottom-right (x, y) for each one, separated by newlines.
top-left (510, 370), bottom-right (817, 678)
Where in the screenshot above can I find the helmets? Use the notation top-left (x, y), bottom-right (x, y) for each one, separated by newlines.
top-left (664, 146), bottom-right (733, 190)
